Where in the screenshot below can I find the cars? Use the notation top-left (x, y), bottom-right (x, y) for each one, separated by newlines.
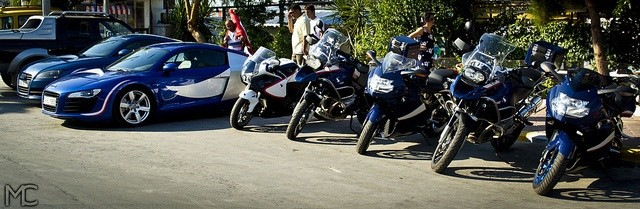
top-left (41, 41), bottom-right (251, 128)
top-left (15, 33), bottom-right (183, 101)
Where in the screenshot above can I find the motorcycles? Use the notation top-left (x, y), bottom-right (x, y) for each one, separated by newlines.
top-left (532, 60), bottom-right (640, 196)
top-left (354, 35), bottom-right (459, 155)
top-left (430, 20), bottom-right (566, 172)
top-left (286, 19), bottom-right (383, 140)
top-left (229, 45), bottom-right (319, 130)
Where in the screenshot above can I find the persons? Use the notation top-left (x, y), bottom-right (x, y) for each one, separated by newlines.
top-left (304, 5), bottom-right (324, 49)
top-left (408, 13), bottom-right (435, 70)
top-left (223, 19), bottom-right (252, 57)
top-left (288, 4), bottom-right (310, 66)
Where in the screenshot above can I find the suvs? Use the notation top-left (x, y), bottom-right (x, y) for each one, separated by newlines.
top-left (0, 4), bottom-right (63, 30)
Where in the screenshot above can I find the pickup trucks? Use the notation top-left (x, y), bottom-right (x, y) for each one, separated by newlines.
top-left (0, 11), bottom-right (137, 90)
